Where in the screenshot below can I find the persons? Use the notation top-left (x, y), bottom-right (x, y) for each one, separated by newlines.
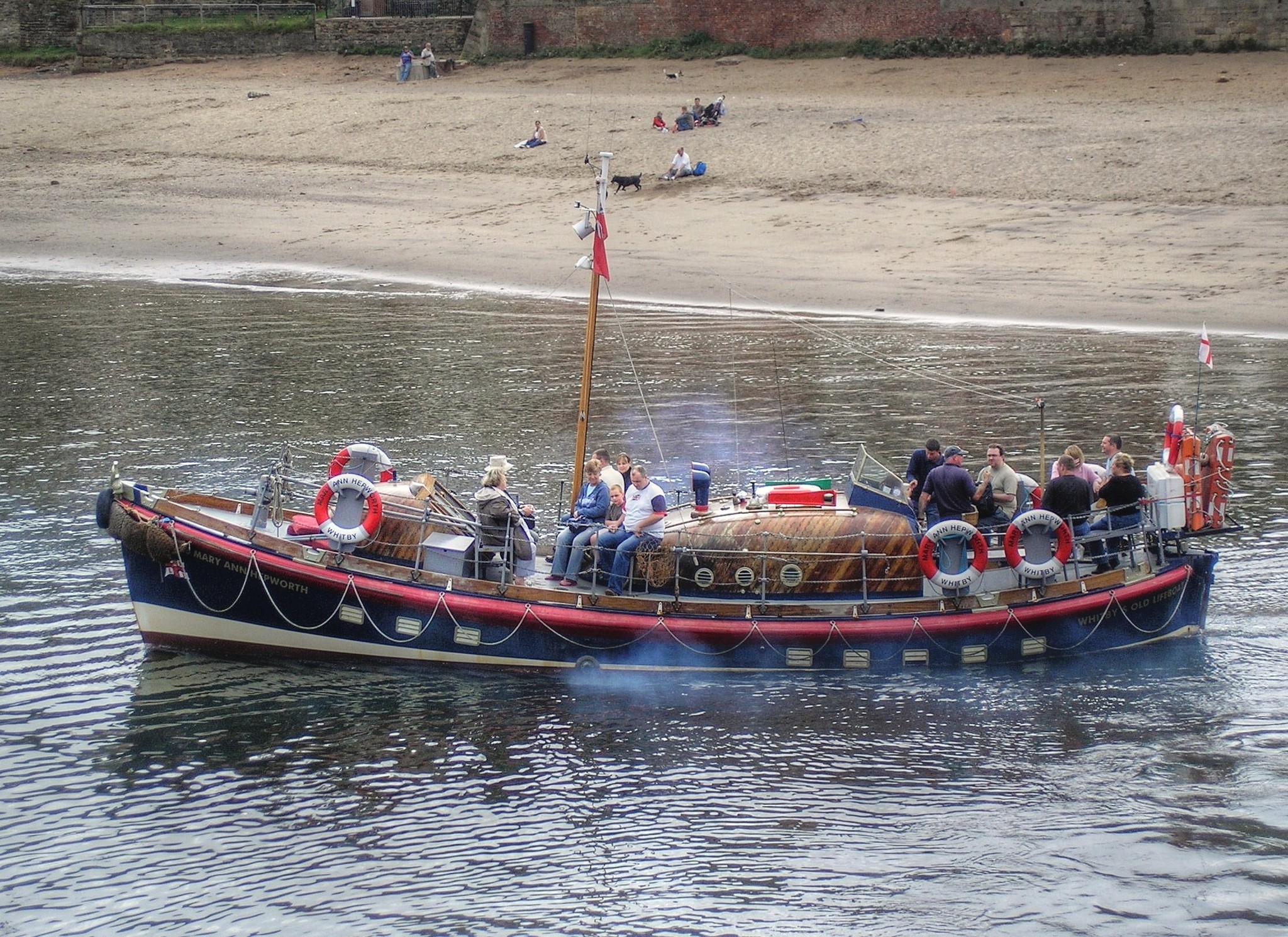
top-left (905, 434), bottom-right (1148, 572)
top-left (471, 447), bottom-right (668, 597)
top-left (400, 42), bottom-right (728, 180)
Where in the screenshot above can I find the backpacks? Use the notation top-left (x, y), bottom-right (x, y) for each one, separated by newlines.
top-left (971, 481), bottom-right (1003, 517)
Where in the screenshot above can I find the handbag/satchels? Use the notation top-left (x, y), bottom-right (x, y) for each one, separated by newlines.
top-left (568, 516), bottom-right (593, 534)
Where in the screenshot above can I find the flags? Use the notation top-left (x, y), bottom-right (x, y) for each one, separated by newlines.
top-left (1198, 323), bottom-right (1212, 369)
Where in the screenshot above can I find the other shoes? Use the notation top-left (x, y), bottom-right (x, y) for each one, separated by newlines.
top-left (545, 555), bottom-right (554, 563)
top-left (1108, 557), bottom-right (1121, 569)
top-left (605, 589), bottom-right (616, 596)
top-left (1091, 562), bottom-right (1110, 574)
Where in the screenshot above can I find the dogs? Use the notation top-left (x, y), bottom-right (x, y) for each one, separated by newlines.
top-left (663, 69), bottom-right (683, 84)
top-left (611, 172), bottom-right (642, 194)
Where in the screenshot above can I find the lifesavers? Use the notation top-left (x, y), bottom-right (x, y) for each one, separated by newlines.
top-left (96, 488), bottom-right (113, 529)
top-left (1163, 404), bottom-right (1183, 472)
top-left (919, 520), bottom-right (987, 589)
top-left (1004, 509), bottom-right (1073, 579)
top-left (314, 473), bottom-right (381, 544)
top-left (327, 443), bottom-right (393, 482)
top-left (1016, 473), bottom-right (1041, 509)
top-left (755, 484), bottom-right (821, 498)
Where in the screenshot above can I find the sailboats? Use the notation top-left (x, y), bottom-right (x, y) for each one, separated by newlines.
top-left (92, 154), bottom-right (1243, 685)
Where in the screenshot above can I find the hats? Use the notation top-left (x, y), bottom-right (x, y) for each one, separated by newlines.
top-left (945, 446), bottom-right (969, 459)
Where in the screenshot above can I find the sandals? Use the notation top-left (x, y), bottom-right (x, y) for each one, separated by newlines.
top-left (560, 578), bottom-right (576, 586)
top-left (545, 573), bottom-right (563, 579)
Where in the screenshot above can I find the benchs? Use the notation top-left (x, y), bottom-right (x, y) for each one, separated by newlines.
top-left (475, 516), bottom-right (514, 583)
top-left (1052, 484), bottom-right (1165, 568)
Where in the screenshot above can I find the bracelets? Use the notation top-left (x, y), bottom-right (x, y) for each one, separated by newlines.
top-left (678, 169), bottom-right (681, 171)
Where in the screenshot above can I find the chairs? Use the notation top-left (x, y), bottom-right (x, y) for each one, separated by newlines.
top-left (997, 481), bottom-right (1032, 546)
